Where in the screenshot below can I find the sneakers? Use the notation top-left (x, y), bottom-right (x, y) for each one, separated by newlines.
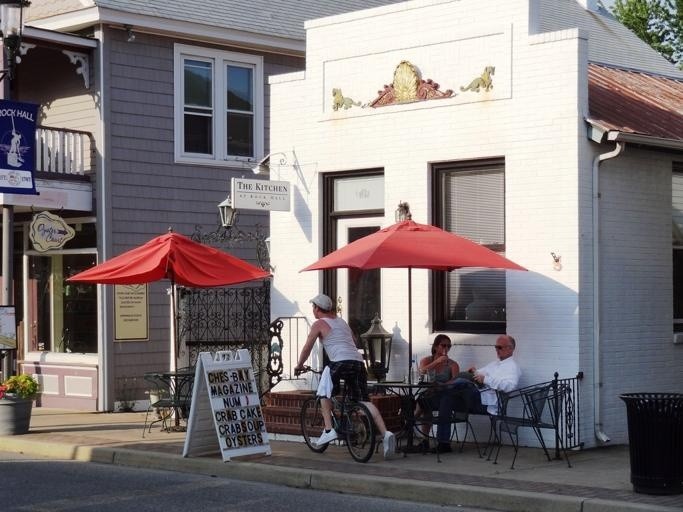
top-left (317, 428), bottom-right (338, 446)
top-left (382, 431), bottom-right (394, 459)
top-left (432, 441), bottom-right (452, 453)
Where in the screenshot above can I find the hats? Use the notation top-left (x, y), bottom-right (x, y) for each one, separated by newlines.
top-left (309, 294), bottom-right (332, 310)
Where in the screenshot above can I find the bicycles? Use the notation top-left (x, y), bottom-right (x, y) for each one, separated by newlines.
top-left (294, 364), bottom-right (375, 462)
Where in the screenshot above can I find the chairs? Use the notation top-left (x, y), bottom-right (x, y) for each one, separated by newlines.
top-left (166, 366), bottom-right (194, 426)
top-left (492, 380), bottom-right (574, 469)
top-left (416, 403), bottom-right (475, 456)
top-left (438, 385), bottom-right (509, 461)
top-left (481, 380), bottom-right (549, 461)
top-left (139, 371), bottom-right (173, 438)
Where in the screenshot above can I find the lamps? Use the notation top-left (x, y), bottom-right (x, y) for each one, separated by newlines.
top-left (359, 310), bottom-right (394, 380)
top-left (393, 200), bottom-right (412, 223)
top-left (216, 193), bottom-right (239, 231)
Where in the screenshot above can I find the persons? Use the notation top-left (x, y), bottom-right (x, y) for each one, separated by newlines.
top-left (413, 334), bottom-right (460, 451)
top-left (295, 294), bottom-right (397, 461)
top-left (431, 335), bottom-right (522, 453)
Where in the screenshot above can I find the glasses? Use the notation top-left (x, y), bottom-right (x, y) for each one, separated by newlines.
top-left (496, 345), bottom-right (502, 349)
top-left (440, 343), bottom-right (452, 348)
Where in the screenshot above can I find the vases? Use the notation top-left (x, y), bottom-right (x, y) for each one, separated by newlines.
top-left (0, 397), bottom-right (33, 435)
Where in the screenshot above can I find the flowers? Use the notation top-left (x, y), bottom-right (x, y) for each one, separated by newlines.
top-left (0, 373), bottom-right (40, 402)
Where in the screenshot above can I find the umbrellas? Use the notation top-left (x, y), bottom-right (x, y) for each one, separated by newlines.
top-left (65, 228), bottom-right (274, 427)
top-left (298, 211), bottom-right (528, 446)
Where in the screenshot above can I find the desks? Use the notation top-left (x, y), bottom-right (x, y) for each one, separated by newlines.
top-left (139, 371), bottom-right (197, 434)
top-left (372, 379), bottom-right (446, 459)
